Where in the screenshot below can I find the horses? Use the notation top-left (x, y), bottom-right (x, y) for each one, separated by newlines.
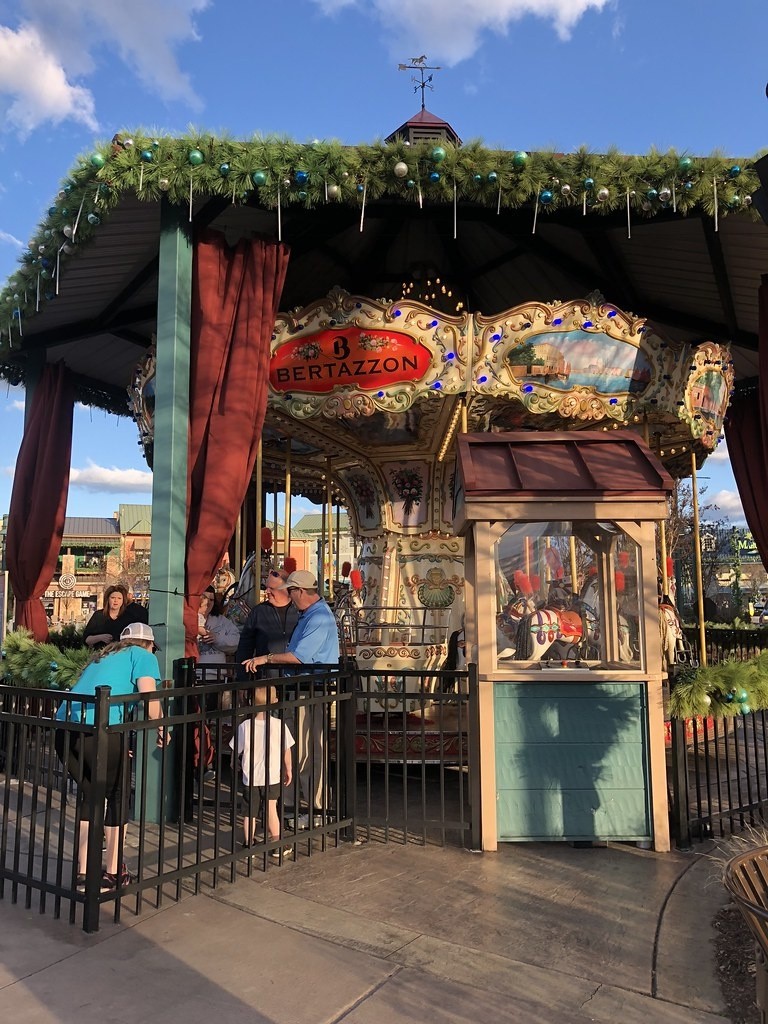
top-left (331, 583), bottom-right (364, 644)
top-left (439, 567), bottom-right (685, 705)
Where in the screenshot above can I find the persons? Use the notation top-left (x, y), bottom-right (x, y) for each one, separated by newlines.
top-left (54, 569), bottom-right (339, 892)
top-left (693, 589), bottom-right (717, 622)
top-left (230, 687), bottom-right (295, 856)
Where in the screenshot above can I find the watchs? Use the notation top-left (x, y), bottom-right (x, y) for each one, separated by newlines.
top-left (268, 653), bottom-right (274, 664)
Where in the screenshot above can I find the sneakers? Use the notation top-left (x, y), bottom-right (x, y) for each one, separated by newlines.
top-left (287, 812), bottom-right (333, 829)
top-left (77, 873), bottom-right (86, 892)
top-left (100, 863), bottom-right (138, 893)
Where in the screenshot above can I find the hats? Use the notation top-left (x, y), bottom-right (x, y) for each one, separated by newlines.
top-left (119, 623), bottom-right (163, 651)
top-left (278, 570), bottom-right (318, 589)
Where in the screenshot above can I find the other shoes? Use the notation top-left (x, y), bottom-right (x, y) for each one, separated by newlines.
top-left (272, 843), bottom-right (292, 857)
top-left (242, 838), bottom-right (256, 859)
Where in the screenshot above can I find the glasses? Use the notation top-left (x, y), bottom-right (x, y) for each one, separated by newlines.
top-left (270, 571), bottom-right (283, 578)
